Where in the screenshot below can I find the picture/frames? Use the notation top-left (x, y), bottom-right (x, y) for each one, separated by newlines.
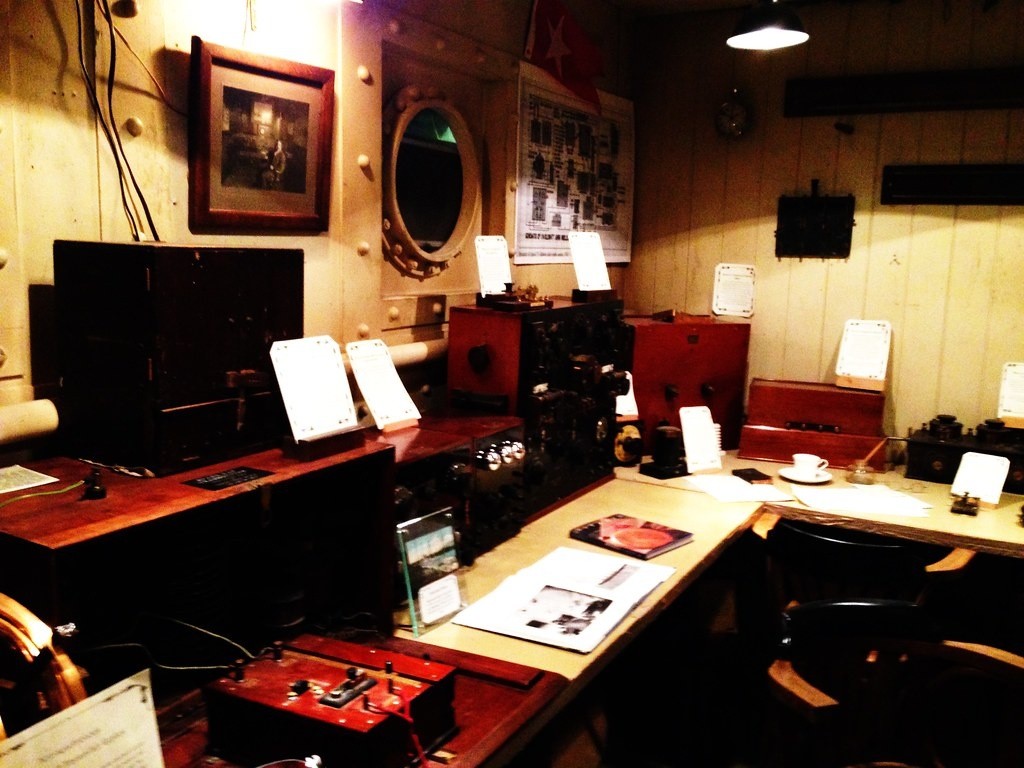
top-left (186, 34), bottom-right (334, 236)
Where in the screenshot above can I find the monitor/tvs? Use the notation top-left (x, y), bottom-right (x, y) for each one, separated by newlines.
top-left (142, 245), bottom-right (305, 455)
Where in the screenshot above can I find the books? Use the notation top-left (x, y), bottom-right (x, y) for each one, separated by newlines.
top-left (398, 507), bottom-right (461, 638)
top-left (732, 468), bottom-right (773, 484)
top-left (569, 514), bottom-right (694, 560)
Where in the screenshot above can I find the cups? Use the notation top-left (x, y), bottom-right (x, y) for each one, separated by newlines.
top-left (792, 453), bottom-right (828, 480)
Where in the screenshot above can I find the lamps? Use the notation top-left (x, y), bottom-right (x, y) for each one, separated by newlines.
top-left (724, 0), bottom-right (810, 54)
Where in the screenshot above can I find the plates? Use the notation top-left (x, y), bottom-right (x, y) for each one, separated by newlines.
top-left (778, 467), bottom-right (832, 483)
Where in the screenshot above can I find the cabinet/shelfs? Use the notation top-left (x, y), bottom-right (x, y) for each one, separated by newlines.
top-left (446, 301), bottom-right (625, 417)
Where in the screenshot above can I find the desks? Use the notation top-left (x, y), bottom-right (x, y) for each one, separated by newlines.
top-left (0, 440), bottom-right (395, 724)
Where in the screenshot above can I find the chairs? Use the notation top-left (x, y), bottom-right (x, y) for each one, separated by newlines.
top-left (770, 521), bottom-right (952, 768)
